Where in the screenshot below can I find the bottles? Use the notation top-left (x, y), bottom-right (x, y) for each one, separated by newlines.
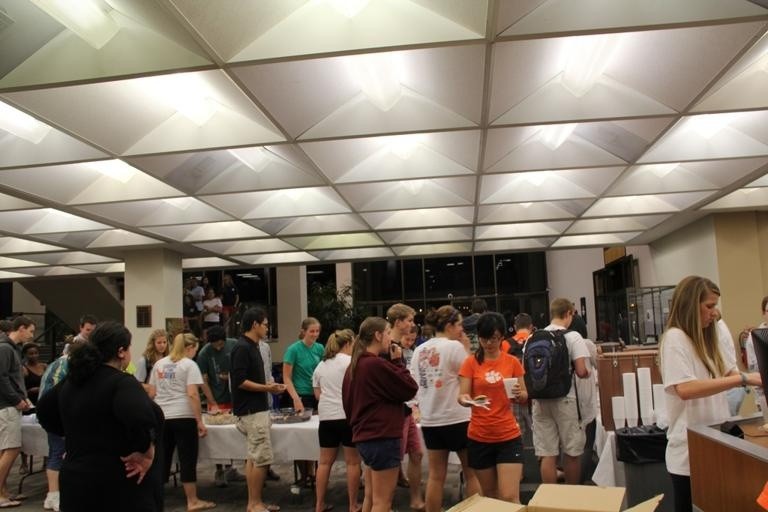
top-left (758, 389), bottom-right (768, 424)
top-left (288, 485), bottom-right (303, 503)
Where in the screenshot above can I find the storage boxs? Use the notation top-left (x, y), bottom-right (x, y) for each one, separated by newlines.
top-left (447, 483), bottom-right (664, 512)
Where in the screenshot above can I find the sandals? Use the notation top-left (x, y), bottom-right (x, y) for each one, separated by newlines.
top-left (0, 498), bottom-right (21, 507)
top-left (8, 494), bottom-right (28, 500)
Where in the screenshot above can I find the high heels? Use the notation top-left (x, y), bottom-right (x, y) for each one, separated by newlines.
top-left (43, 492), bottom-right (60, 512)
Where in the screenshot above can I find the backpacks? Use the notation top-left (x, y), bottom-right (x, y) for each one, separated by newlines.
top-left (523, 329), bottom-right (576, 398)
top-left (507, 336), bottom-right (525, 357)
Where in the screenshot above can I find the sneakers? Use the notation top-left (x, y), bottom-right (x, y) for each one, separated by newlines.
top-left (224, 468), bottom-right (246, 481)
top-left (215, 473), bottom-right (228, 486)
top-left (268, 470), bottom-right (280, 480)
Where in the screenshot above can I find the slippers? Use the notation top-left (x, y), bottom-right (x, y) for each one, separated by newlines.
top-left (323, 504), bottom-right (334, 512)
top-left (264, 503), bottom-right (280, 511)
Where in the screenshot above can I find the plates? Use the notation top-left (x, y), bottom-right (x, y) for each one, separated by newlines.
top-left (469, 401), bottom-right (489, 406)
top-left (758, 423), bottom-right (768, 431)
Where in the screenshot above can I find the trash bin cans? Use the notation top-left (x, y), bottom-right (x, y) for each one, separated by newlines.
top-left (615, 426), bottom-right (676, 512)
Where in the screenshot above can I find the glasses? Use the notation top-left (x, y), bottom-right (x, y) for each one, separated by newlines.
top-left (481, 335), bottom-right (499, 342)
top-left (261, 322), bottom-right (268, 329)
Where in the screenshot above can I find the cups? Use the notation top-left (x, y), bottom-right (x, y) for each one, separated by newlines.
top-left (637, 367), bottom-right (653, 425)
top-left (652, 384), bottom-right (666, 427)
top-left (612, 397), bottom-right (626, 430)
top-left (622, 373), bottom-right (639, 429)
top-left (504, 378), bottom-right (518, 399)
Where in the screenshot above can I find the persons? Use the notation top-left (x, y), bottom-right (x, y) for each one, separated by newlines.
top-left (744, 294), bottom-right (768, 414)
top-left (283, 297), bottom-right (599, 512)
top-left (1, 274), bottom-right (288, 512)
top-left (711, 306), bottom-right (746, 418)
top-left (654, 275), bottom-right (764, 512)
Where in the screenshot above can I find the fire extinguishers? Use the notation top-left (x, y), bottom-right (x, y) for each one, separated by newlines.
top-left (739, 326), bottom-right (754, 369)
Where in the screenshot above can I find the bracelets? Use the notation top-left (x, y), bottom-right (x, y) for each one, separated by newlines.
top-left (740, 372), bottom-right (748, 386)
